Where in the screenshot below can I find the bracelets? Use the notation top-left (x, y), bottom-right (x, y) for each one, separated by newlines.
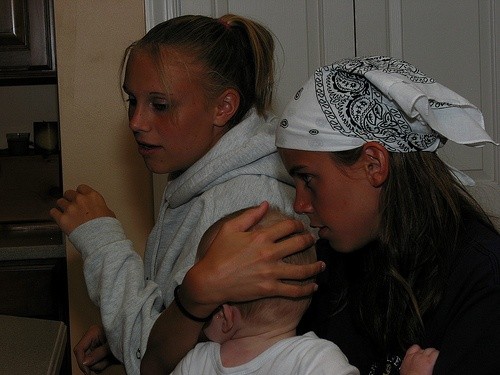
top-left (174, 284), bottom-right (214, 323)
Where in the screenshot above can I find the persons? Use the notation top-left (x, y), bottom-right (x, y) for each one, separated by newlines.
top-left (142, 56), bottom-right (500, 374)
top-left (50, 15), bottom-right (320, 374)
top-left (171, 205), bottom-right (438, 374)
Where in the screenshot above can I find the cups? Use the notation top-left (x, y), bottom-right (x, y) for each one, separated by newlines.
top-left (6, 133), bottom-right (30, 149)
top-left (33, 121), bottom-right (58, 150)
